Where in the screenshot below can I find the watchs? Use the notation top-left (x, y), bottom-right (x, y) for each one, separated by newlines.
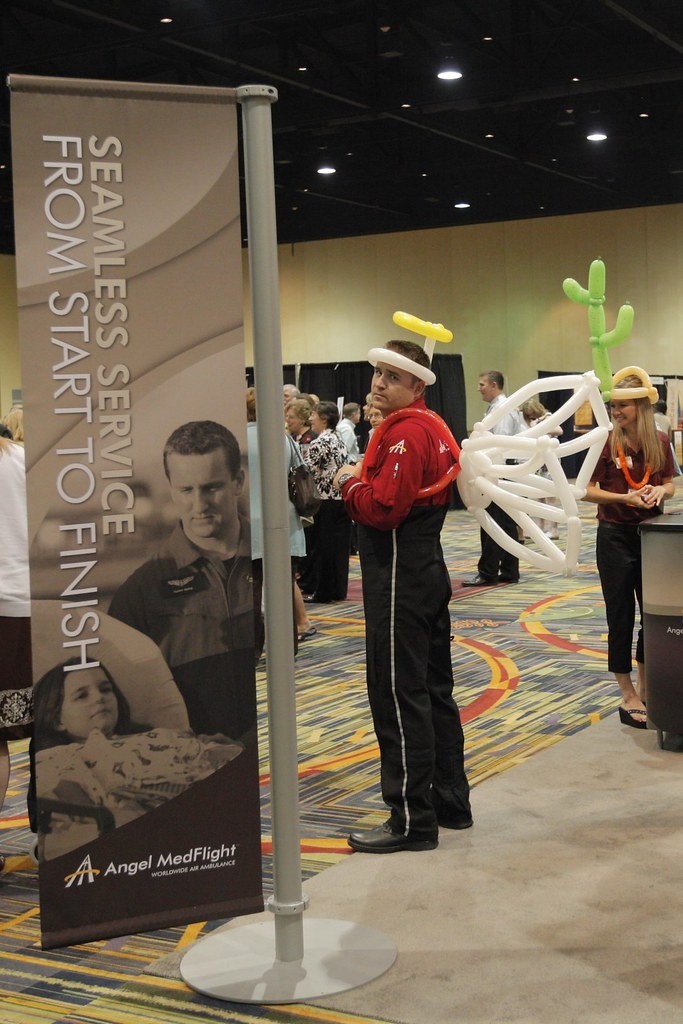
top-left (338, 473), bottom-right (355, 489)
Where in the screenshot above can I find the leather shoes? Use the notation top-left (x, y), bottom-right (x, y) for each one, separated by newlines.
top-left (347, 821), bottom-right (438, 854)
top-left (461, 574), bottom-right (497, 587)
top-left (498, 574), bottom-right (518, 583)
top-left (303, 593), bottom-right (331, 602)
top-left (438, 810), bottom-right (474, 830)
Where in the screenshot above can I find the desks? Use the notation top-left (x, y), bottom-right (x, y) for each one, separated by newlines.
top-left (638, 508), bottom-right (683, 751)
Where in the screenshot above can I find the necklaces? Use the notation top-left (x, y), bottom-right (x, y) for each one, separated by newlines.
top-left (619, 434), bottom-right (651, 488)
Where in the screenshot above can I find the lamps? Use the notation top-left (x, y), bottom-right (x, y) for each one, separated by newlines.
top-left (435, 42), bottom-right (463, 81)
top-left (586, 103), bottom-right (607, 141)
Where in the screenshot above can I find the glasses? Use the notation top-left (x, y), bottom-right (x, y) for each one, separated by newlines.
top-left (366, 412), bottom-right (380, 419)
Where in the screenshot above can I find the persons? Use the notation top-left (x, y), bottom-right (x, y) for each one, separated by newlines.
top-left (462, 370), bottom-right (522, 587)
top-left (35, 655), bottom-right (160, 749)
top-left (362, 392), bottom-right (386, 446)
top-left (246, 383), bottom-right (349, 643)
top-left (514, 399), bottom-right (565, 544)
top-left (335, 402), bottom-right (360, 554)
top-left (108, 421), bottom-right (255, 739)
top-left (331, 340), bottom-right (475, 854)
top-left (0, 422), bottom-right (41, 866)
top-left (580, 367), bottom-right (681, 730)
top-left (304, 401), bottom-right (348, 606)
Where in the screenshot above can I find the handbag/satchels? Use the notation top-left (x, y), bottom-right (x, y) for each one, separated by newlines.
top-left (288, 464), bottom-right (323, 518)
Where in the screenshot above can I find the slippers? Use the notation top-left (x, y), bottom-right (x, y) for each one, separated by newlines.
top-left (619, 706), bottom-right (648, 729)
top-left (298, 627), bottom-right (318, 641)
